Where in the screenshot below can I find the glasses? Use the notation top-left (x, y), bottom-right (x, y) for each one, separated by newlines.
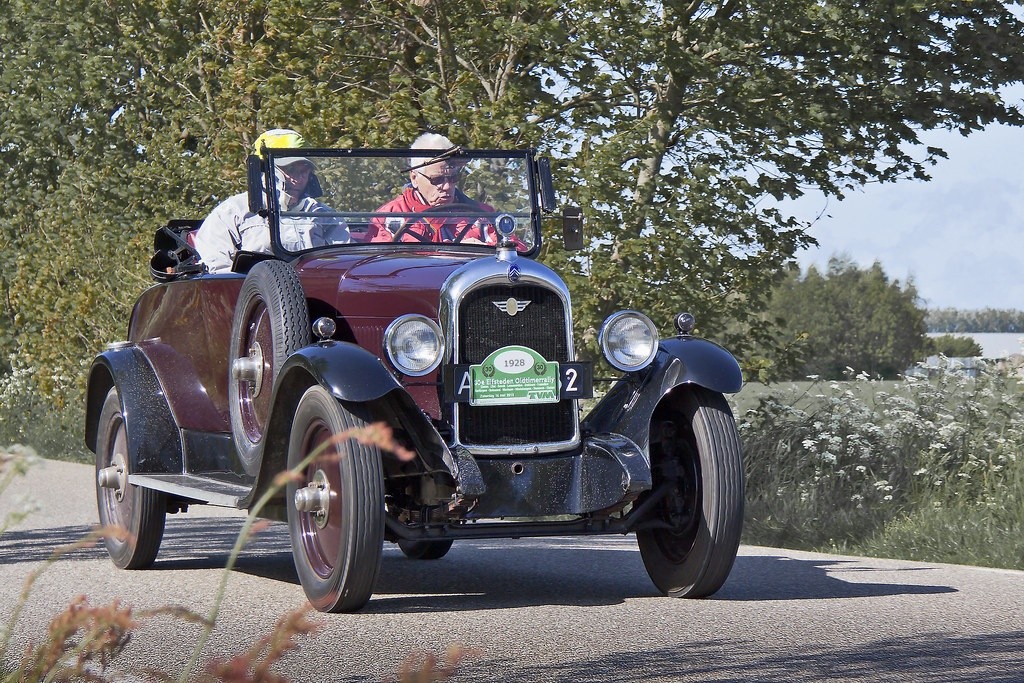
top-left (416, 172), bottom-right (462, 185)
top-left (282, 166), bottom-right (310, 178)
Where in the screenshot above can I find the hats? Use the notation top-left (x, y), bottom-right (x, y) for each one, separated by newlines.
top-left (250, 128), bottom-right (318, 172)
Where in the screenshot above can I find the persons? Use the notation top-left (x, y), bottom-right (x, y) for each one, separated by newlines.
top-left (366, 134), bottom-right (527, 251)
top-left (195, 128), bottom-right (351, 274)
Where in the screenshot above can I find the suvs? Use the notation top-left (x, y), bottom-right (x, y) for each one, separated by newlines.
top-left (81, 146), bottom-right (749, 620)
